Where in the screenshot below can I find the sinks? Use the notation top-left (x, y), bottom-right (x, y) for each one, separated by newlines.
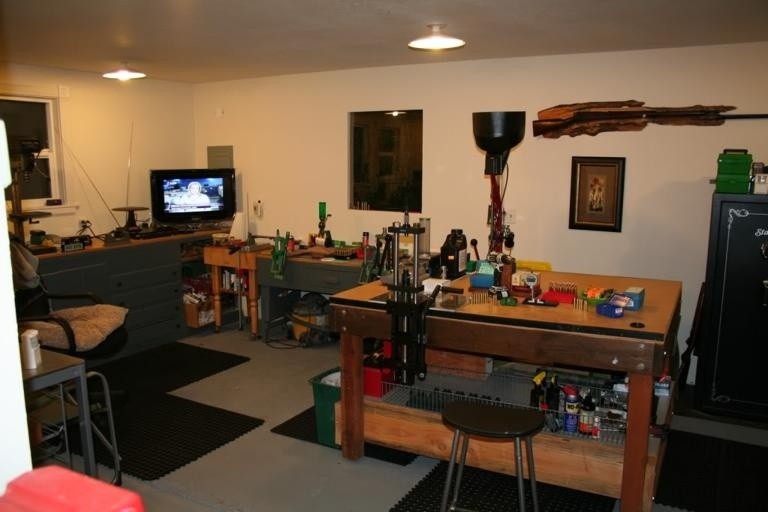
top-left (287, 252), bottom-right (334, 260)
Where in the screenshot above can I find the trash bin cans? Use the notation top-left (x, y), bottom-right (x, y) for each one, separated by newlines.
top-left (308, 364), bottom-right (342, 450)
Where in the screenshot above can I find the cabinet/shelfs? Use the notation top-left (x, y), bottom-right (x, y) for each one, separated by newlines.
top-left (691, 191), bottom-right (768, 421)
top-left (26, 226), bottom-right (243, 371)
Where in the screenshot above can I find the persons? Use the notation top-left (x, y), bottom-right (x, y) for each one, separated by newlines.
top-left (177, 181), bottom-right (213, 204)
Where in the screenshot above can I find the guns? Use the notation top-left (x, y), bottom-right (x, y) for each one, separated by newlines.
top-left (533, 111), bottom-right (767, 136)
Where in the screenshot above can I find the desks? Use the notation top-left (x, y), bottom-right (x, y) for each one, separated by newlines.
top-left (203, 244), bottom-right (409, 341)
top-left (326, 270), bottom-right (682, 512)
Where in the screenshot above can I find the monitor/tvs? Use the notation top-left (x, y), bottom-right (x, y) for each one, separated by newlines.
top-left (150, 168), bottom-right (236, 231)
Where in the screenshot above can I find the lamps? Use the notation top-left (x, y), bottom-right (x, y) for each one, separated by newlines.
top-left (407, 23), bottom-right (466, 52)
top-left (101, 61), bottom-right (147, 82)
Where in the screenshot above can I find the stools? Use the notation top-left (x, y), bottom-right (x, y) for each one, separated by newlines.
top-left (440, 401), bottom-right (545, 512)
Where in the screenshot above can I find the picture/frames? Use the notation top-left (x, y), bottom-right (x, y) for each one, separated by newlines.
top-left (568, 155), bottom-right (626, 232)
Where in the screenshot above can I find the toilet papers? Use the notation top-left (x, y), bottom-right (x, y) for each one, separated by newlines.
top-left (230, 212), bottom-right (247, 244)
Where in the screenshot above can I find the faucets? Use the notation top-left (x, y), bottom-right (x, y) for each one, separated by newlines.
top-left (317, 221), bottom-right (331, 248)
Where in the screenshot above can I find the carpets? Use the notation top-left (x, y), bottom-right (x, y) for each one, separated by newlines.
top-left (270, 404), bottom-right (420, 466)
top-left (653, 426), bottom-right (767, 512)
top-left (47, 341), bottom-right (266, 480)
top-left (389, 460), bottom-right (618, 512)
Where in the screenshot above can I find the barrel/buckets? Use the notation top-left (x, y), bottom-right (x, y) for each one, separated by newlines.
top-left (441, 228), bottom-right (468, 280)
